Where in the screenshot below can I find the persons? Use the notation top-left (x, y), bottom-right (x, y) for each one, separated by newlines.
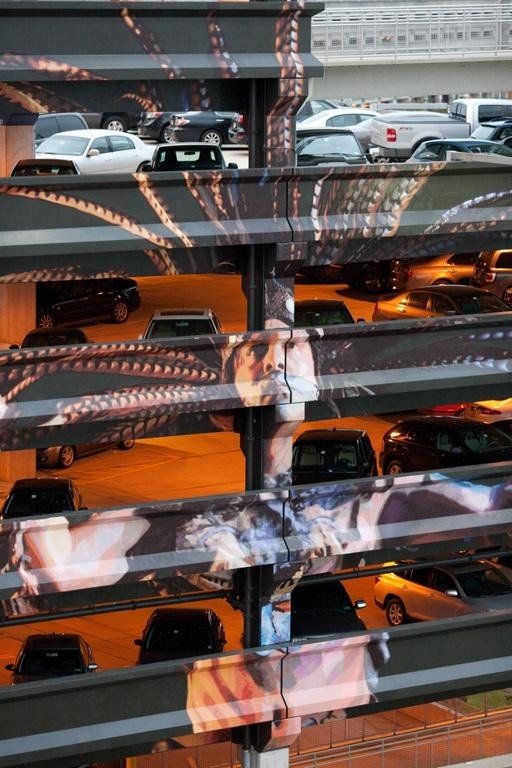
top-left (217, 329), bottom-right (318, 403)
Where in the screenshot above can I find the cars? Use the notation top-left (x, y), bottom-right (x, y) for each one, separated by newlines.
top-left (37, 434), bottom-right (137, 466)
top-left (136, 607), bottom-right (228, 666)
top-left (1, 478), bottom-right (92, 519)
top-left (464, 546), bottom-right (512, 584)
top-left (37, 274), bottom-right (141, 328)
top-left (406, 397), bottom-right (512, 435)
top-left (292, 248), bottom-right (512, 327)
top-left (6, 632), bottom-right (98, 688)
top-left (8, 326), bottom-right (96, 351)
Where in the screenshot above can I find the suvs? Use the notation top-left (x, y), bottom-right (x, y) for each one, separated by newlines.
top-left (291, 427), bottom-right (380, 483)
top-left (241, 571), bottom-right (367, 650)
top-left (372, 552), bottom-right (510, 628)
top-left (140, 305), bottom-right (224, 344)
top-left (379, 416), bottom-right (511, 476)
